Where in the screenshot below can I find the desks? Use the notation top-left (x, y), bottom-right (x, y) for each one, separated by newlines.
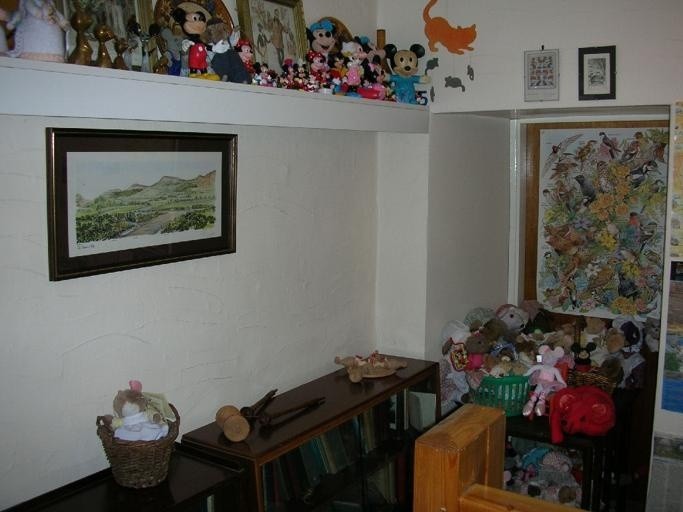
top-left (0, 440), bottom-right (248, 512)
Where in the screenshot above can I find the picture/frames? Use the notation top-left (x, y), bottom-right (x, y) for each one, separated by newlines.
top-left (45, 127), bottom-right (238, 281)
top-left (522, 45), bottom-right (616, 102)
top-left (235, 0), bottom-right (307, 73)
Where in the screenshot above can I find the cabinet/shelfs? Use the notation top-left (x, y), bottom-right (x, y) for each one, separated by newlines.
top-left (180, 352), bottom-right (441, 511)
top-left (453, 386), bottom-right (640, 512)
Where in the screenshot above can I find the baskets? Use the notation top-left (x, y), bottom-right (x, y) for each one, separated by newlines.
top-left (473, 376), bottom-right (531, 417)
top-left (96, 403), bottom-right (180, 488)
top-left (566, 370), bottom-right (617, 397)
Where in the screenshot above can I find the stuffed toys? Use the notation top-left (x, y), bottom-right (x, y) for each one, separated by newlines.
top-left (439, 297), bottom-right (661, 510)
top-left (0, 0), bottom-right (431, 105)
top-left (441, 297), bottom-right (660, 509)
